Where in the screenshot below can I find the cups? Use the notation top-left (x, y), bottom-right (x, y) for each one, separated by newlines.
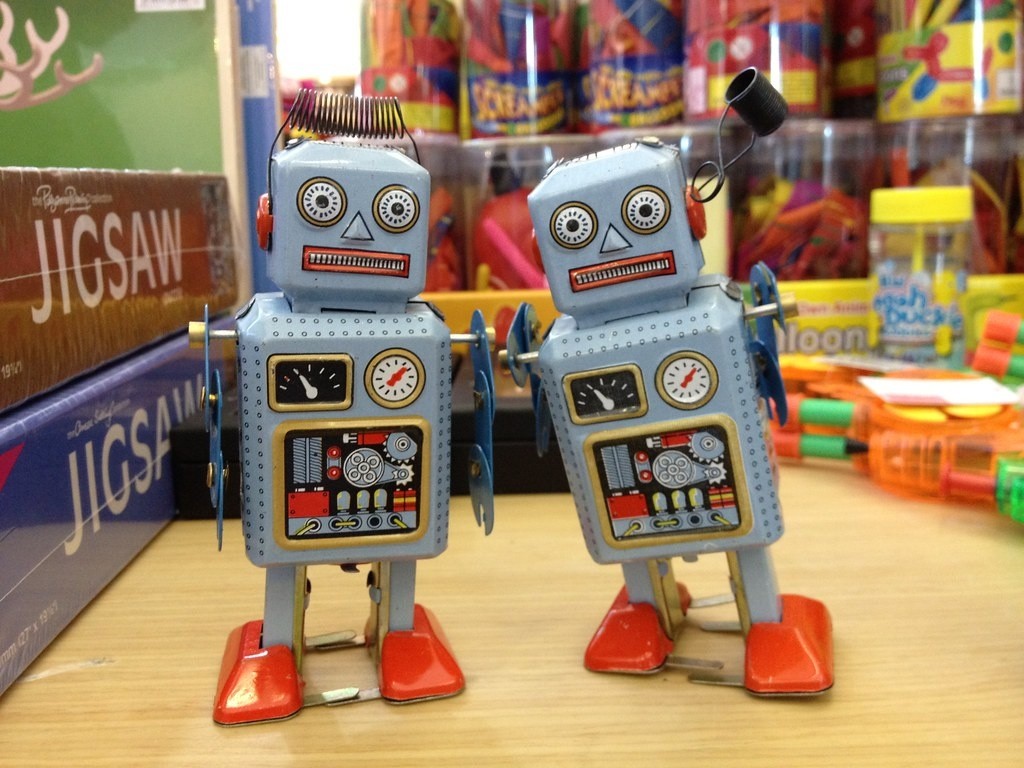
top-left (359, 0), bottom-right (1024, 295)
top-left (867, 183), bottom-right (980, 371)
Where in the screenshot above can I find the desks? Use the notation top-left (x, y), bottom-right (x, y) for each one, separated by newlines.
top-left (0, 463), bottom-right (1024, 768)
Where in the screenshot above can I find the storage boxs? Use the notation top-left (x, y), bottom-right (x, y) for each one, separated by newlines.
top-left (0, 312), bottom-right (234, 699)
top-left (0, 166), bottom-right (237, 415)
